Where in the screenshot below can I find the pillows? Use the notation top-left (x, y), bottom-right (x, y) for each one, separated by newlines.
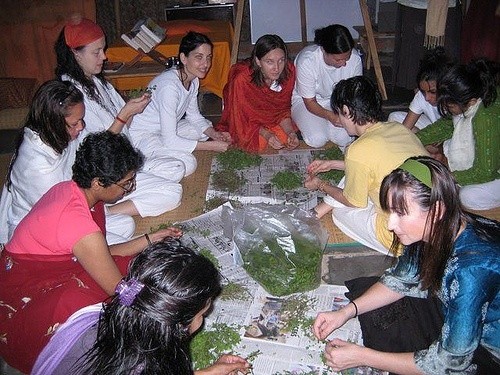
top-left (159, 20), bottom-right (210, 36)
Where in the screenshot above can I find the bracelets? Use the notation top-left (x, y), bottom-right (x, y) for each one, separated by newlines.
top-left (319, 181), bottom-right (326, 191)
top-left (349, 300), bottom-right (357, 318)
top-left (144, 234), bottom-right (152, 246)
top-left (115, 117), bottom-right (126, 123)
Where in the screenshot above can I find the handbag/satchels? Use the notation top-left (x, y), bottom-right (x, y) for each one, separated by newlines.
top-left (222, 204), bottom-right (328, 296)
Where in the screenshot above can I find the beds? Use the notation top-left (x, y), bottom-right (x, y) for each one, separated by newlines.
top-left (104, 19), bottom-right (233, 98)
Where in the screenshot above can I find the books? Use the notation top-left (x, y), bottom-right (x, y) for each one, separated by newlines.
top-left (99, 19), bottom-right (167, 73)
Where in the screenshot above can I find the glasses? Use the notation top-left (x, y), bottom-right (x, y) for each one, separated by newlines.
top-left (114, 178), bottom-right (133, 193)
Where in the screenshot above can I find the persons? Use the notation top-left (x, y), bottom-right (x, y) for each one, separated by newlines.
top-left (0, 131), bottom-right (183, 375)
top-left (213, 34), bottom-right (301, 152)
top-left (311, 156), bottom-right (500, 375)
top-left (305, 49), bottom-right (500, 258)
top-left (292, 24), bottom-right (364, 146)
top-left (0, 80), bottom-right (137, 245)
top-left (51, 17), bottom-right (187, 216)
top-left (30, 233), bottom-right (252, 375)
top-left (134, 31), bottom-right (233, 154)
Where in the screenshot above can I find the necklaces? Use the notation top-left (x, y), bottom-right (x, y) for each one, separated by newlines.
top-left (179, 68), bottom-right (191, 92)
